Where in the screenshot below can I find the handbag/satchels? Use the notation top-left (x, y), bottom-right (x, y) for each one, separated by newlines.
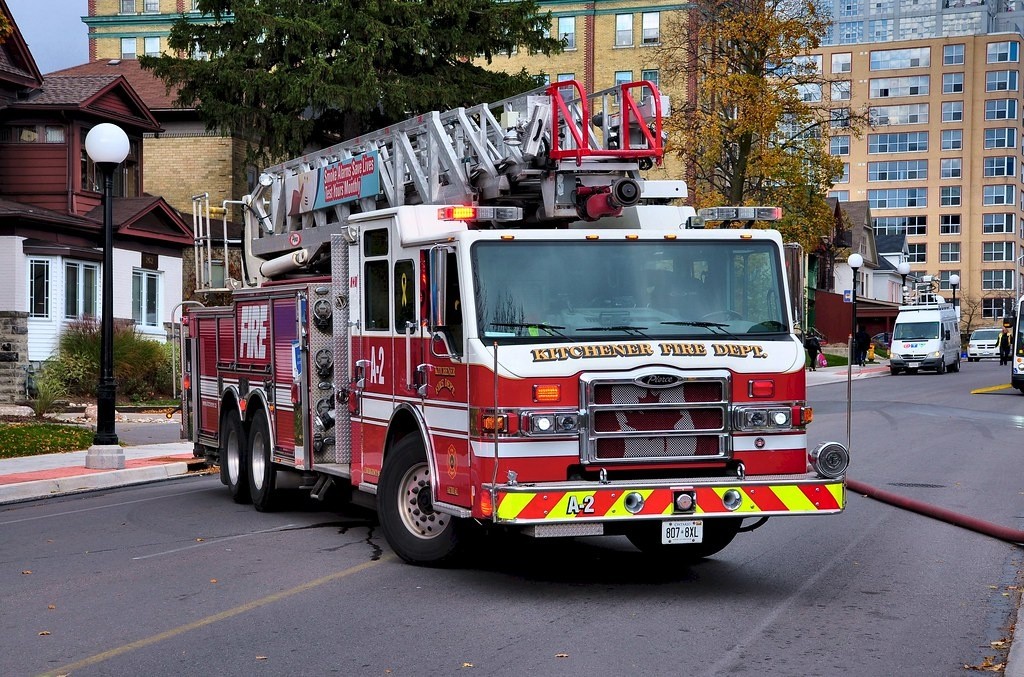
top-left (817, 353), bottom-right (827, 367)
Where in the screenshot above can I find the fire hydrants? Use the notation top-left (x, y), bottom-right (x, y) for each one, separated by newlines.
top-left (867, 345), bottom-right (874, 363)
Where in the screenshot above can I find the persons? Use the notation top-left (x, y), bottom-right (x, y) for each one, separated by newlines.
top-left (855, 326), bottom-right (871, 367)
top-left (804, 332), bottom-right (822, 372)
top-left (995, 327), bottom-right (1013, 366)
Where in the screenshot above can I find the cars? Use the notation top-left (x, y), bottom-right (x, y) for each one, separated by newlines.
top-left (870, 333), bottom-right (892, 348)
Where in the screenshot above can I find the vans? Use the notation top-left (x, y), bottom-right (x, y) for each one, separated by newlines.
top-left (966, 328), bottom-right (1012, 362)
top-left (884, 275), bottom-right (963, 375)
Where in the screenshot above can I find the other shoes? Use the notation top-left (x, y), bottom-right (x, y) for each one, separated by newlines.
top-left (859, 363), bottom-right (861, 366)
top-left (809, 369), bottom-right (811, 371)
top-left (813, 370), bottom-right (816, 371)
top-left (863, 363), bottom-right (865, 366)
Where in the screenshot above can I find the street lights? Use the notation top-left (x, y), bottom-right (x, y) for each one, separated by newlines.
top-left (83, 122), bottom-right (131, 470)
top-left (950, 274), bottom-right (961, 312)
top-left (847, 253), bottom-right (864, 366)
top-left (897, 262), bottom-right (911, 306)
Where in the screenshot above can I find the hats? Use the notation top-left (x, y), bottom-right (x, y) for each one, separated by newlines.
top-left (805, 331), bottom-right (811, 335)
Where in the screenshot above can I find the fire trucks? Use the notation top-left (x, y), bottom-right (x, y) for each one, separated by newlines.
top-left (170, 78), bottom-right (853, 569)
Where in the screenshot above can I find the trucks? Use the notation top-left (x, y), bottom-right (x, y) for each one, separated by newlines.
top-left (1002, 295), bottom-right (1024, 393)
